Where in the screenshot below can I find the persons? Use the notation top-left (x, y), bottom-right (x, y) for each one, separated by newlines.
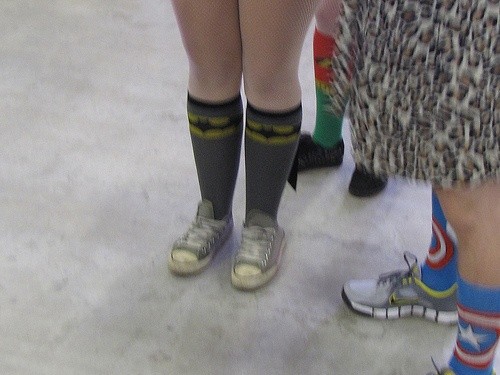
top-left (297, 0), bottom-right (389, 197)
top-left (326, 0), bottom-right (500, 375)
top-left (170, 0), bottom-right (318, 292)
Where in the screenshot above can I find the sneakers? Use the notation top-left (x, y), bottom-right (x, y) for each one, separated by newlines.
top-left (232, 209), bottom-right (286, 289)
top-left (295, 133), bottom-right (344, 171)
top-left (169, 199), bottom-right (234, 277)
top-left (342, 251), bottom-right (458, 325)
top-left (348, 163), bottom-right (388, 197)
